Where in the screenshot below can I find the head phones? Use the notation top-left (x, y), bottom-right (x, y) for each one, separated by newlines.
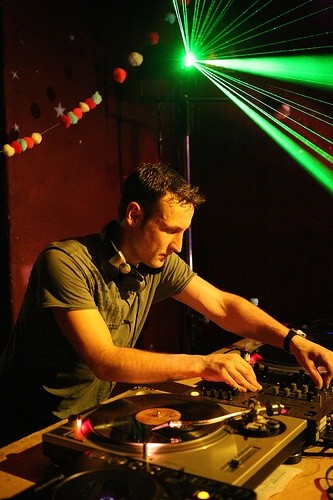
top-left (99, 219), bottom-right (165, 293)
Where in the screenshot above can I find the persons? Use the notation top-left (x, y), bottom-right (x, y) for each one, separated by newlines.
top-left (0, 160), bottom-right (333, 432)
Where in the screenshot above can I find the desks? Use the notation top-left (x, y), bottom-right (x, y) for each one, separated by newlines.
top-left (0, 338), bottom-right (333, 500)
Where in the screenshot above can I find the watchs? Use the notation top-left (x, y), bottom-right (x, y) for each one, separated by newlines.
top-left (283, 326), bottom-right (307, 356)
